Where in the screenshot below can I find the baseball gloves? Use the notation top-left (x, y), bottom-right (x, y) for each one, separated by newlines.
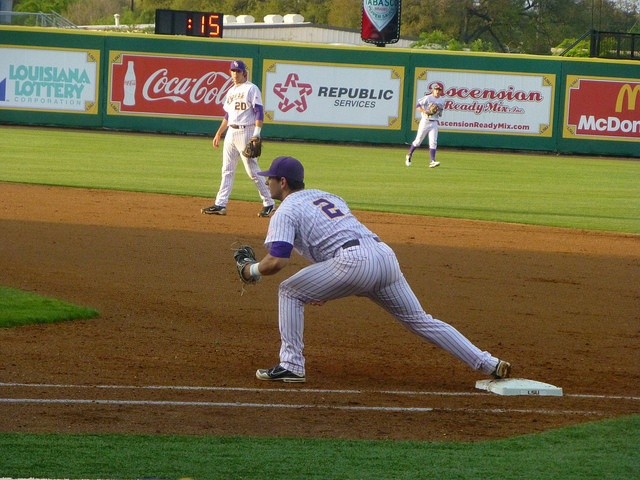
top-left (426, 103), bottom-right (439, 116)
top-left (241, 137), bottom-right (262, 158)
top-left (234, 245), bottom-right (260, 285)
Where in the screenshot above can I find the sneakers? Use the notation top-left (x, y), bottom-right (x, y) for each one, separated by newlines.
top-left (489, 361), bottom-right (511, 383)
top-left (201, 206), bottom-right (226, 215)
top-left (256, 366), bottom-right (306, 383)
top-left (258, 206), bottom-right (274, 218)
top-left (429, 160), bottom-right (440, 167)
top-left (405, 155), bottom-right (412, 167)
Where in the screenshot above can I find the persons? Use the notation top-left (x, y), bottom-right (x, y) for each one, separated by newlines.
top-left (406, 85), bottom-right (444, 167)
top-left (201, 61), bottom-right (276, 217)
top-left (233, 156), bottom-right (513, 383)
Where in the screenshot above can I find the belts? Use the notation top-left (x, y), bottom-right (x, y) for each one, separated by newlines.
top-left (230, 125), bottom-right (246, 130)
top-left (342, 237), bottom-right (381, 249)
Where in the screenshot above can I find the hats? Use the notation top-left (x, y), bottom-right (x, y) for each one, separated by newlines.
top-left (230, 60), bottom-right (246, 71)
top-left (432, 83), bottom-right (443, 90)
top-left (256, 157), bottom-right (304, 187)
